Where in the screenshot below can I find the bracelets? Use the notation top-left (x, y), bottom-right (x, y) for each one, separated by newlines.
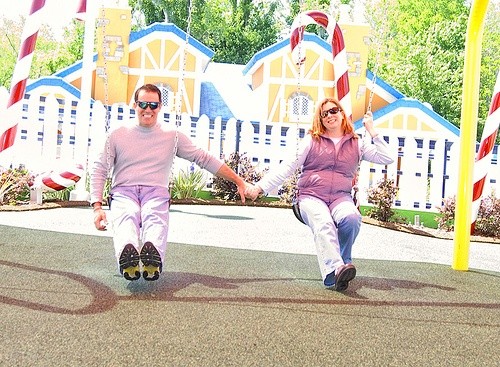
top-left (93, 206), bottom-right (102, 212)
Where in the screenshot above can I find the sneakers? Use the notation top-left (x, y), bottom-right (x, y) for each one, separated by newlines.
top-left (118, 243), bottom-right (140, 280)
top-left (140, 240), bottom-right (161, 281)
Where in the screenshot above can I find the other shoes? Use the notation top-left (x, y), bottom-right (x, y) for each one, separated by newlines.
top-left (334, 264), bottom-right (356, 291)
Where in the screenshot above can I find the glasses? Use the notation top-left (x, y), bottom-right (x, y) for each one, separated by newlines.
top-left (320, 106), bottom-right (340, 117)
top-left (136, 100), bottom-right (160, 110)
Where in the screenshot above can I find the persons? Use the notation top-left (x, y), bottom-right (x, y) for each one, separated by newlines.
top-left (90, 84), bottom-right (260, 281)
top-left (244, 98), bottom-right (395, 291)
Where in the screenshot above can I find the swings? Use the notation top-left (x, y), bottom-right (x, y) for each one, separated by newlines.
top-left (292, 0), bottom-right (386, 226)
top-left (101, 0), bottom-right (193, 227)
